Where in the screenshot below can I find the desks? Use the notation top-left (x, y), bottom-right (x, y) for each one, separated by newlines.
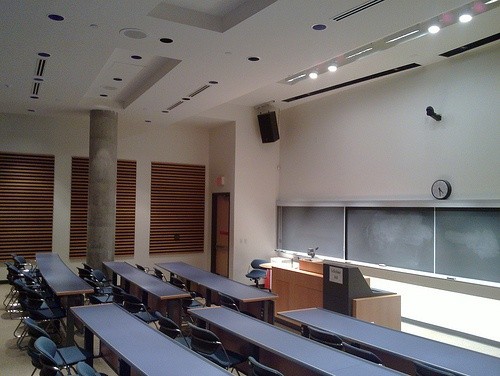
top-left (277, 305), bottom-right (499, 376)
top-left (269, 268), bottom-right (402, 333)
top-left (154, 261), bottom-right (279, 322)
top-left (35, 253), bottom-right (95, 336)
top-left (186, 305), bottom-right (407, 376)
top-left (102, 261), bottom-right (192, 327)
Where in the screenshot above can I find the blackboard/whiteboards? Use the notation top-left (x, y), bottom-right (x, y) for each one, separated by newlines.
top-left (276, 202), bottom-right (500, 288)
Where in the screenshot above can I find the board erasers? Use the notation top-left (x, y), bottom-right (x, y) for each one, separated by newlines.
top-left (379, 264), bottom-right (386, 266)
top-left (447, 276), bottom-right (455, 280)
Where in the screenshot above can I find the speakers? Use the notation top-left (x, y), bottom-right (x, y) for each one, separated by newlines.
top-left (257, 111), bottom-right (280, 143)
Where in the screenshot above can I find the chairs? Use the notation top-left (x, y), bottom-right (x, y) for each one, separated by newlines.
top-left (70, 302), bottom-right (233, 375)
top-left (0, 253), bottom-right (449, 376)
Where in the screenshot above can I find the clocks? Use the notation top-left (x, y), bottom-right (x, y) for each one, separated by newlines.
top-left (431, 179), bottom-right (451, 199)
